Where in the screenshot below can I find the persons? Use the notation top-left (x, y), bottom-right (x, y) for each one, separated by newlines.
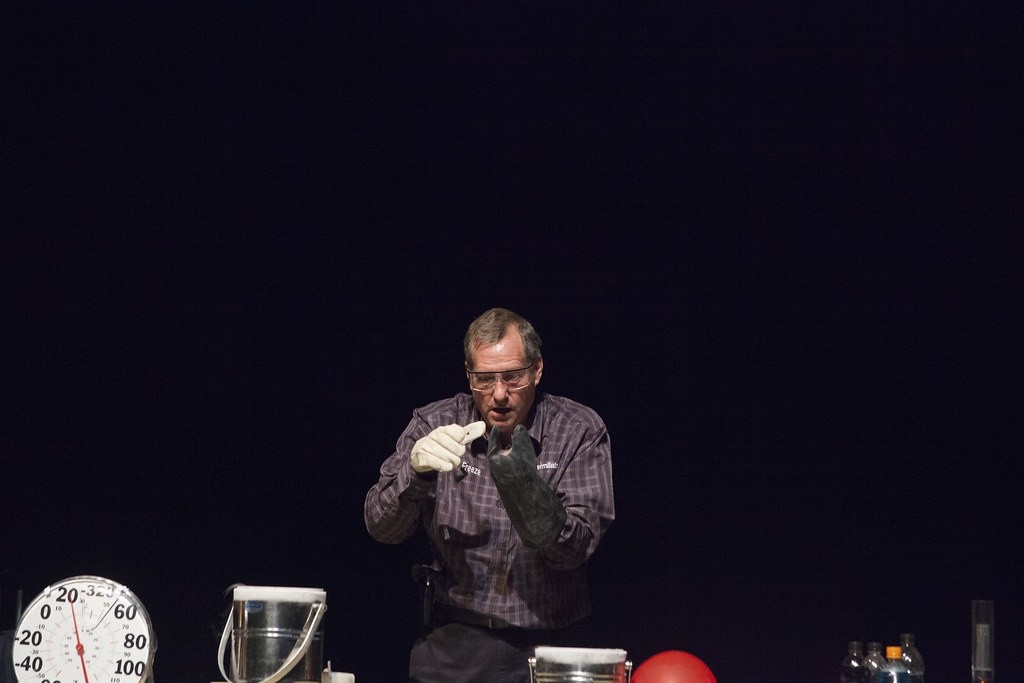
top-left (364, 307), bottom-right (616, 683)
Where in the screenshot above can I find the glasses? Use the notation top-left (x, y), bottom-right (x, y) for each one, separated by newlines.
top-left (467, 364), bottom-right (533, 391)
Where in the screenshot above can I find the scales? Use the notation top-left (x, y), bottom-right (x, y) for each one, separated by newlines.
top-left (11, 574), bottom-right (157, 683)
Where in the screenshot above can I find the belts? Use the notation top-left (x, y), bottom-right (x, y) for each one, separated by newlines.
top-left (433, 603), bottom-right (513, 629)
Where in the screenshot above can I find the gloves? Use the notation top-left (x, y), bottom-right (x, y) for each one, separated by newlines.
top-left (487, 424), bottom-right (567, 550)
top-left (410, 420), bottom-right (486, 474)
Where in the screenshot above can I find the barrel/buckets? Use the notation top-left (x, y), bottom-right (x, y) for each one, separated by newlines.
top-left (218, 585), bottom-right (327, 683)
top-left (527, 644), bottom-right (633, 683)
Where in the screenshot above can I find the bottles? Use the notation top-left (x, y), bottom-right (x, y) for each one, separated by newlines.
top-left (839, 641), bottom-right (865, 683)
top-left (900, 634), bottom-right (926, 683)
top-left (877, 646), bottom-right (911, 683)
top-left (860, 642), bottom-right (887, 683)
